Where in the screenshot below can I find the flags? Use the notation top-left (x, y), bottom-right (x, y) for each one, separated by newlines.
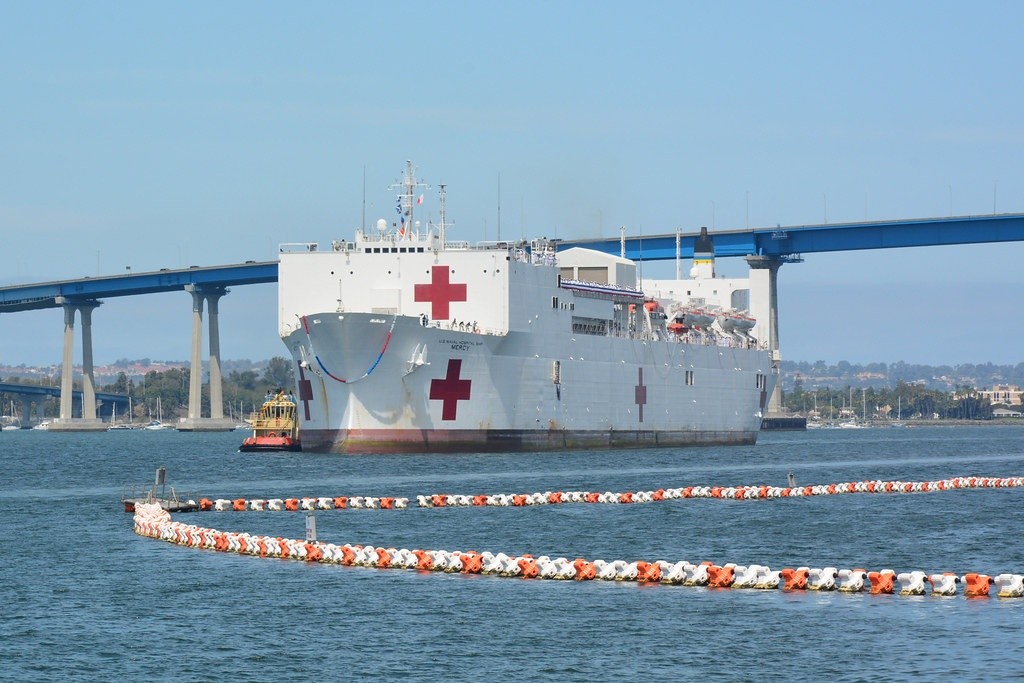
top-left (415, 193), bottom-right (423, 205)
top-left (396, 197), bottom-right (404, 234)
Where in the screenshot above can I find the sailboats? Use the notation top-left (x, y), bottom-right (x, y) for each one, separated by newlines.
top-left (807, 396), bottom-right (834, 428)
top-left (109, 402), bottom-right (134, 430)
top-left (145, 397), bottom-right (166, 430)
top-left (0, 400), bottom-right (21, 430)
top-left (892, 396), bottom-right (905, 427)
top-left (228, 401), bottom-right (241, 429)
top-left (839, 387), bottom-right (864, 428)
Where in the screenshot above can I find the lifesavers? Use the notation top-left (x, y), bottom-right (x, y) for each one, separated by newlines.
top-left (268, 432), bottom-right (276, 437)
top-left (475, 329), bottom-right (481, 335)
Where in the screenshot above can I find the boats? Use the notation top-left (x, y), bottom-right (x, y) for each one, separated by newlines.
top-left (674, 306), bottom-right (716, 326)
top-left (239, 391), bottom-right (302, 453)
top-left (717, 309), bottom-right (756, 331)
top-left (34, 421), bottom-right (51, 430)
top-left (278, 159), bottom-right (780, 456)
